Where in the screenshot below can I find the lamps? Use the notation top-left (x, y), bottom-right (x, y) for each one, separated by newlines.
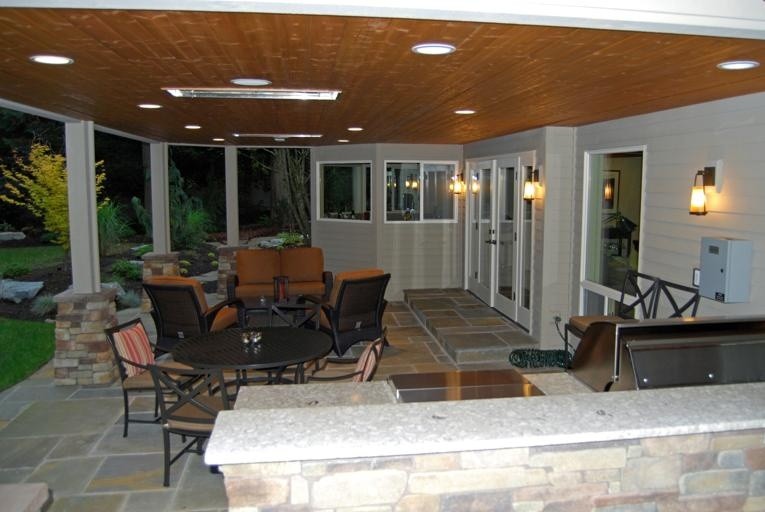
top-left (688, 155), bottom-right (718, 217)
top-left (521, 169), bottom-right (540, 200)
top-left (404, 174), bottom-right (418, 191)
top-left (451, 173), bottom-right (464, 197)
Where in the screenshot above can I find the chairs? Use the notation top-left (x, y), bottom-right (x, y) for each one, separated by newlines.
top-left (104, 303), bottom-right (387, 487)
top-left (564, 265), bottom-right (699, 354)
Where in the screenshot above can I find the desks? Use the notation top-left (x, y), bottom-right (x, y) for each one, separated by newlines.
top-left (238, 295), bottom-right (320, 328)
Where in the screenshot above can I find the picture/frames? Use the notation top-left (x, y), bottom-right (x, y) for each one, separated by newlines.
top-left (598, 169), bottom-right (621, 216)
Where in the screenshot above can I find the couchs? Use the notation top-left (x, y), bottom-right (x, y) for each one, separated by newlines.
top-left (142, 276), bottom-right (247, 364)
top-left (295, 269), bottom-right (392, 358)
top-left (227, 247), bottom-right (333, 309)
